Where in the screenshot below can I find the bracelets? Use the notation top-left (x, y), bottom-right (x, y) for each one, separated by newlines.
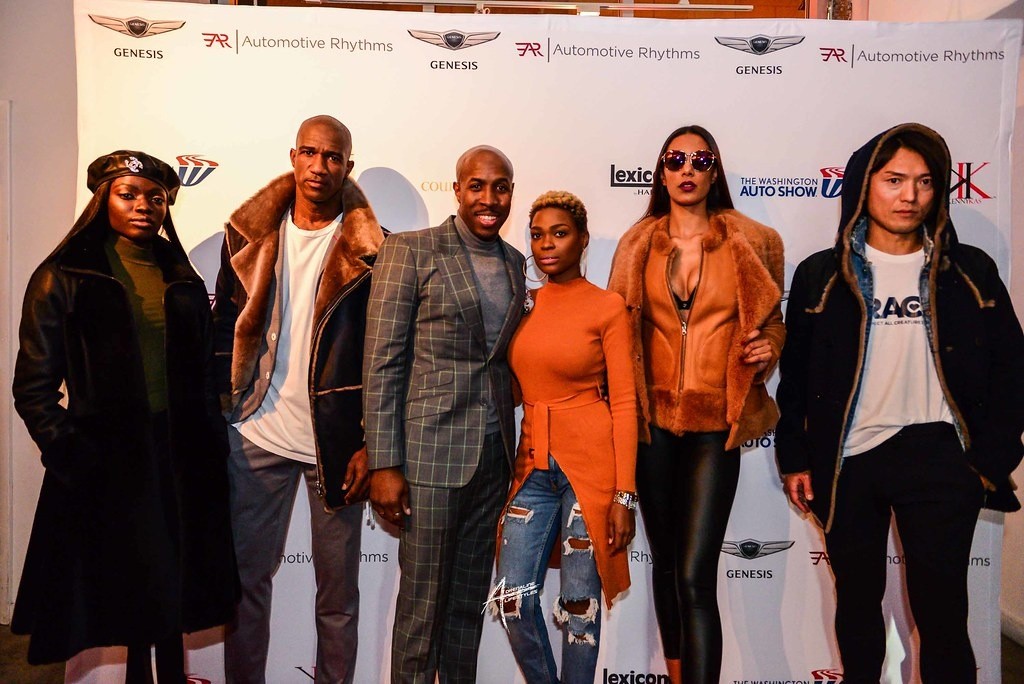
top-left (613, 491), bottom-right (639, 511)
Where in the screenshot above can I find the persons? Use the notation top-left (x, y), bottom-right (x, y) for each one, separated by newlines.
top-left (480, 190), bottom-right (644, 684)
top-left (363, 145), bottom-right (529, 684)
top-left (11, 150), bottom-right (242, 684)
top-left (773, 123), bottom-right (1024, 684)
top-left (606, 125), bottom-right (787, 684)
top-left (208, 114), bottom-right (394, 684)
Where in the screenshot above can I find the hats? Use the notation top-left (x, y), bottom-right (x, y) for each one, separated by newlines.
top-left (87, 150), bottom-right (180, 206)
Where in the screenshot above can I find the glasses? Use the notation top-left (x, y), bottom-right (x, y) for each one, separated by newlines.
top-left (659, 149), bottom-right (717, 173)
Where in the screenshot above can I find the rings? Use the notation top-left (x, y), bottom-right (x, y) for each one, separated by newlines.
top-left (393, 511), bottom-right (402, 517)
top-left (378, 512), bottom-right (385, 516)
